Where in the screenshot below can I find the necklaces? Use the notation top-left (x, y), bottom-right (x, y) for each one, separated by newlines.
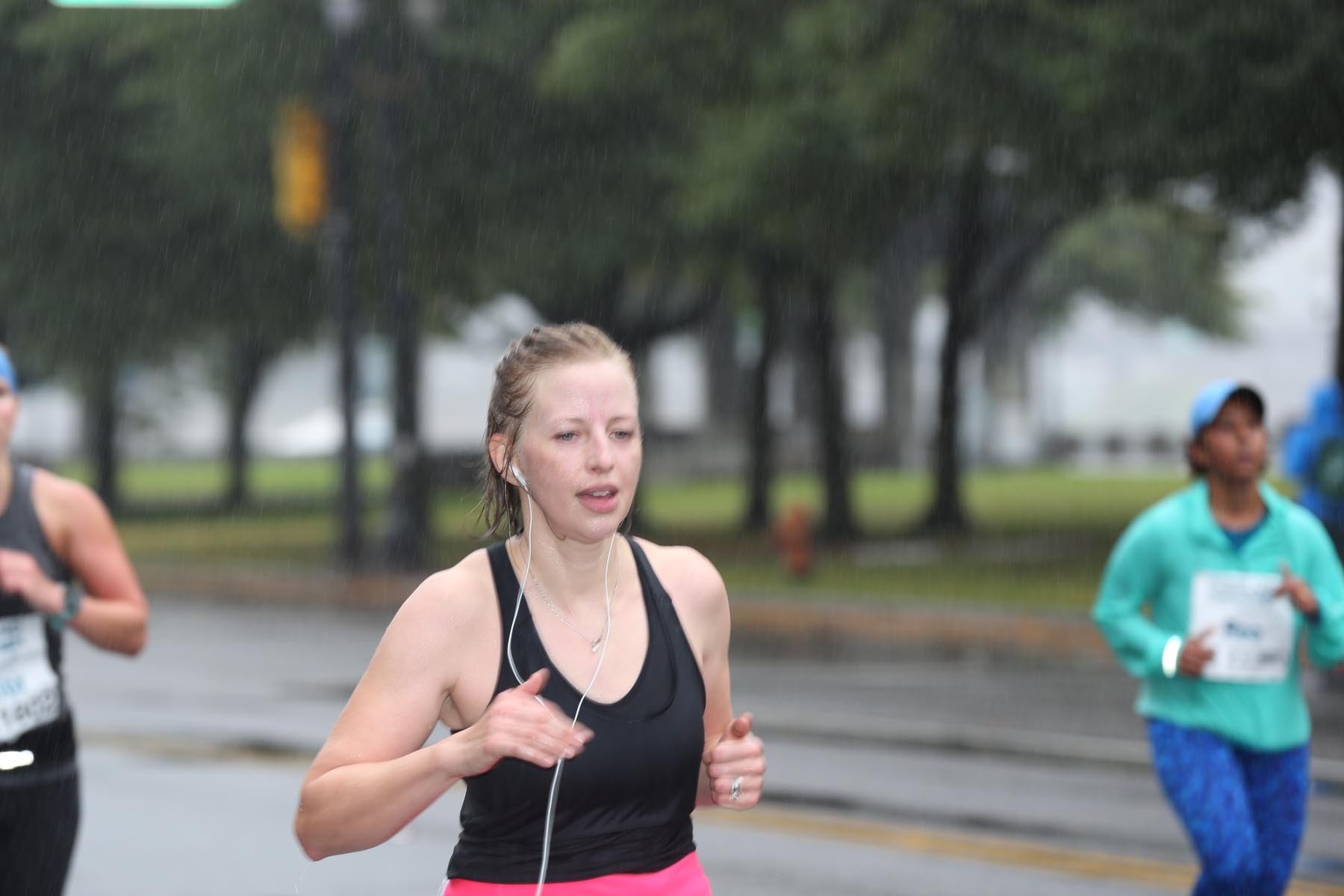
top-left (519, 538), bottom-right (622, 653)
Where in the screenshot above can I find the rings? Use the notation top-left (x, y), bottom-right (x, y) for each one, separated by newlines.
top-left (730, 778), bottom-right (740, 801)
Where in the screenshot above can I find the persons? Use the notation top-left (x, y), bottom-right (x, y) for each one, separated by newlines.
top-left (1090, 376), bottom-right (1343, 896)
top-left (293, 323), bottom-right (769, 896)
top-left (1, 347), bottom-right (149, 896)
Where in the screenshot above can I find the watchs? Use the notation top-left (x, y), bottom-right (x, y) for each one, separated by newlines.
top-left (49, 581), bottom-right (82, 630)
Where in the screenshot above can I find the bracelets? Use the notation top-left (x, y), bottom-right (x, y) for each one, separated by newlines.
top-left (1163, 636), bottom-right (1183, 678)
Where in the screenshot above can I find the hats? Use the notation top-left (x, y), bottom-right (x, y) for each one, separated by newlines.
top-left (1190, 375), bottom-right (1266, 442)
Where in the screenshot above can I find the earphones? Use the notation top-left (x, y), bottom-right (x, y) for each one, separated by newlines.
top-left (509, 464), bottom-right (526, 487)
